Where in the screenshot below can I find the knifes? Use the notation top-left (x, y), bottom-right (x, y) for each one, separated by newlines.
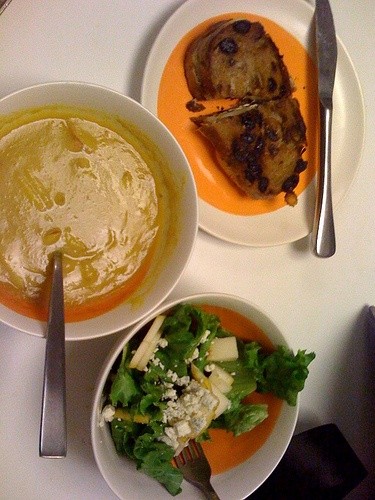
top-left (313, 1), bottom-right (337, 255)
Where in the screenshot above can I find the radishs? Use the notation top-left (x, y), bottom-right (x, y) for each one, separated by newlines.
top-left (187, 379), bottom-right (220, 411)
top-left (212, 364), bottom-right (235, 385)
top-left (168, 391), bottom-right (215, 446)
top-left (208, 372), bottom-right (233, 393)
top-left (129, 314), bottom-right (168, 370)
top-left (208, 335), bottom-right (240, 362)
top-left (135, 332), bottom-right (163, 371)
top-left (189, 362), bottom-right (230, 420)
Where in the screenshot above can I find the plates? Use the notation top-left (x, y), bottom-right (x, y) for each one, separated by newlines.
top-left (141, 1), bottom-right (365, 247)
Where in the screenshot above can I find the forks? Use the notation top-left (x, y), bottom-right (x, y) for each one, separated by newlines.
top-left (174, 437), bottom-right (221, 500)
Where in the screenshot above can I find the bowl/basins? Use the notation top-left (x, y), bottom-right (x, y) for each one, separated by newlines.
top-left (91, 294), bottom-right (300, 500)
top-left (0, 81), bottom-right (197, 342)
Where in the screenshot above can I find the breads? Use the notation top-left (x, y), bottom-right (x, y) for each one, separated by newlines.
top-left (183, 17), bottom-right (292, 100)
top-left (178, 101), bottom-right (323, 201)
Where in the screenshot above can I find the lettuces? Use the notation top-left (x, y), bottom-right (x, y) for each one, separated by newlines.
top-left (110, 301), bottom-right (316, 496)
